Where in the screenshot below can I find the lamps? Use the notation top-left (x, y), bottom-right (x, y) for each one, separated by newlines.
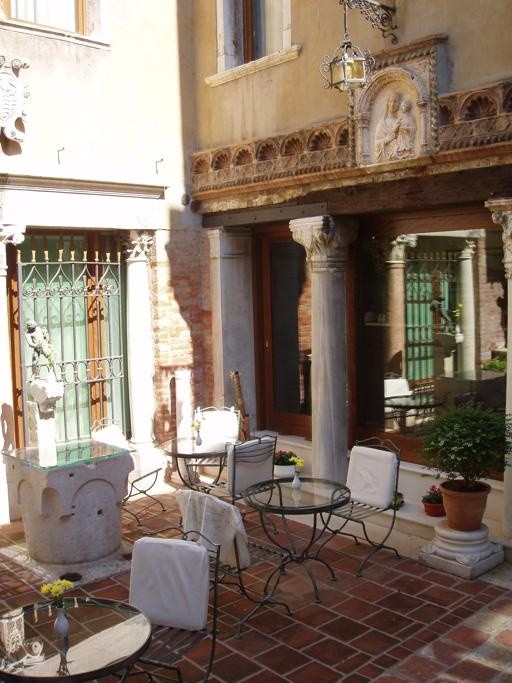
top-left (317, 0), bottom-right (399, 98)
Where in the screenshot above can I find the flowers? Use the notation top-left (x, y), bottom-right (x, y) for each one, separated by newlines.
top-left (45, 348), bottom-right (58, 370)
top-left (287, 454), bottom-right (306, 474)
top-left (188, 418), bottom-right (204, 433)
top-left (39, 575), bottom-right (75, 607)
top-left (421, 483), bottom-right (446, 505)
top-left (55, 662), bottom-right (73, 680)
top-left (450, 302), bottom-right (466, 321)
top-left (272, 449), bottom-right (296, 465)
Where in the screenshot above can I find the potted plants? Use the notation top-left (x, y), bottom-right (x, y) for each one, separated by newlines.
top-left (412, 392), bottom-right (512, 534)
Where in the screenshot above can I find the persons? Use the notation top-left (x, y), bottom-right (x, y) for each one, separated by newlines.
top-left (393, 100), bottom-right (417, 156)
top-left (374, 93), bottom-right (402, 163)
top-left (25, 320), bottom-right (64, 383)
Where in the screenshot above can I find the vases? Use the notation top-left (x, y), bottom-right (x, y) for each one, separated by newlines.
top-left (272, 465), bottom-right (296, 478)
top-left (52, 605), bottom-right (70, 638)
top-left (291, 472), bottom-right (303, 491)
top-left (420, 497), bottom-right (446, 518)
top-left (56, 637), bottom-right (70, 660)
top-left (194, 430), bottom-right (204, 445)
top-left (45, 366), bottom-right (59, 384)
top-left (454, 322), bottom-right (461, 334)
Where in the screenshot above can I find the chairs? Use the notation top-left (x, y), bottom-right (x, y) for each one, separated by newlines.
top-left (172, 487), bottom-right (297, 640)
top-left (383, 369), bottom-right (411, 432)
top-left (185, 404), bottom-right (243, 496)
top-left (90, 415), bottom-right (168, 526)
top-left (196, 432), bottom-right (280, 540)
top-left (314, 434), bottom-right (403, 578)
top-left (106, 525), bottom-right (225, 681)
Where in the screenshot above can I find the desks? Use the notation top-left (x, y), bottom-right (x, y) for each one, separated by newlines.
top-left (157, 432), bottom-right (242, 530)
top-left (2, 435), bottom-right (139, 566)
top-left (241, 474), bottom-right (354, 607)
top-left (0, 591), bottom-right (156, 682)
top-left (382, 393), bottom-right (446, 433)
